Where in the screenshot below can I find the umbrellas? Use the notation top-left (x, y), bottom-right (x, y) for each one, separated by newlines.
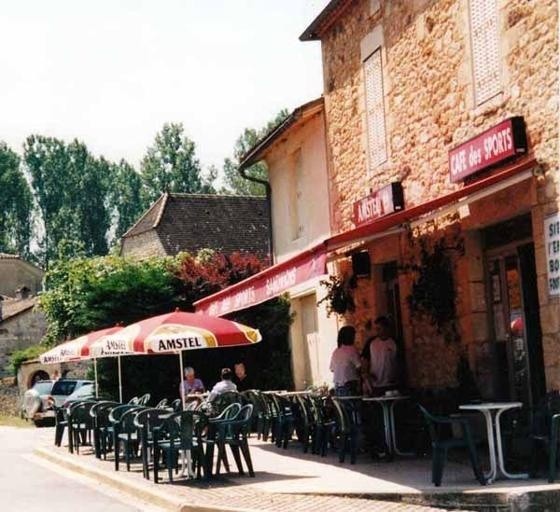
top-left (61, 307), bottom-right (263, 411)
top-left (39, 323), bottom-right (180, 404)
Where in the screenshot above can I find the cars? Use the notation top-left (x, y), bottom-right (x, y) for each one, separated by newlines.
top-left (62, 385), bottom-right (94, 422)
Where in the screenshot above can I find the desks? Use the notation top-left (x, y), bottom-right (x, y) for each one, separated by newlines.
top-left (460, 401), bottom-right (528, 482)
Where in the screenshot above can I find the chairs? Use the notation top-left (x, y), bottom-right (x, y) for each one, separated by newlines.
top-left (418, 403), bottom-right (486, 485)
top-left (263, 391), bottom-right (413, 464)
top-left (50, 387), bottom-right (263, 485)
top-left (528, 406), bottom-right (560, 484)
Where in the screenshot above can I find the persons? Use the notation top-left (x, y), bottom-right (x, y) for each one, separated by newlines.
top-left (207, 368), bottom-right (237, 402)
top-left (234, 361), bottom-right (257, 392)
top-left (180, 366), bottom-right (205, 397)
top-left (329, 326), bottom-right (363, 395)
top-left (360, 316), bottom-right (398, 394)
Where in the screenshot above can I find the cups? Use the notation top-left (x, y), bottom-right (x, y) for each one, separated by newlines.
top-left (385, 391), bottom-right (393, 396)
top-left (393, 390), bottom-right (399, 395)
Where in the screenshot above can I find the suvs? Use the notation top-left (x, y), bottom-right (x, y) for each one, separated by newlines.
top-left (24, 378), bottom-right (95, 427)
